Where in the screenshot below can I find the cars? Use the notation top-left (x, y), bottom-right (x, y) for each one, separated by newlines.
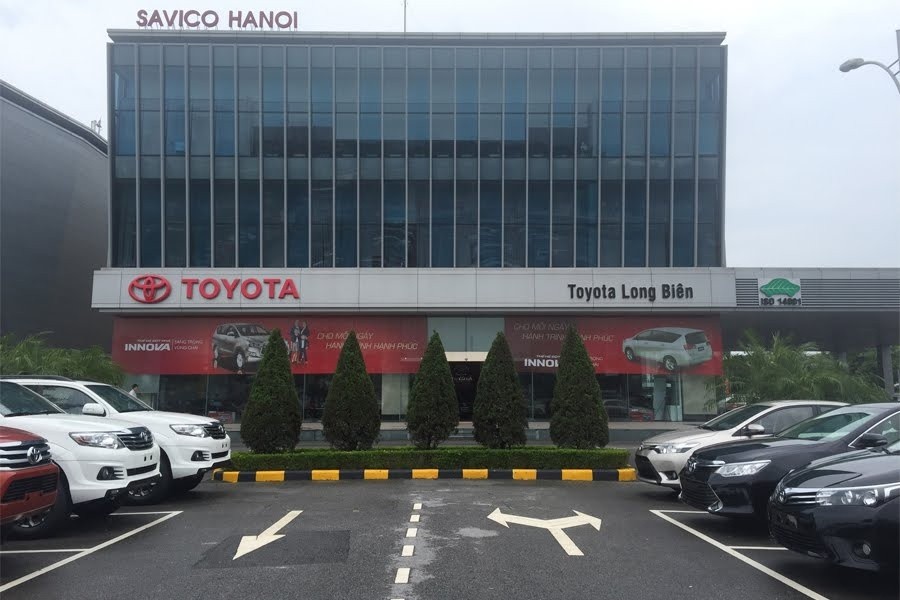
top-left (602, 400), bottom-right (637, 418)
top-left (635, 401), bottom-right (900, 574)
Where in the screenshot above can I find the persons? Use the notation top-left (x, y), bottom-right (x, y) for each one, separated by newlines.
top-left (288, 319), bottom-right (311, 365)
top-left (128, 384), bottom-right (139, 410)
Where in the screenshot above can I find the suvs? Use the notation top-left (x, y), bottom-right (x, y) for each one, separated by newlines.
top-left (213, 323), bottom-right (291, 372)
top-left (0, 376), bottom-right (232, 540)
top-left (623, 327), bottom-right (711, 372)
